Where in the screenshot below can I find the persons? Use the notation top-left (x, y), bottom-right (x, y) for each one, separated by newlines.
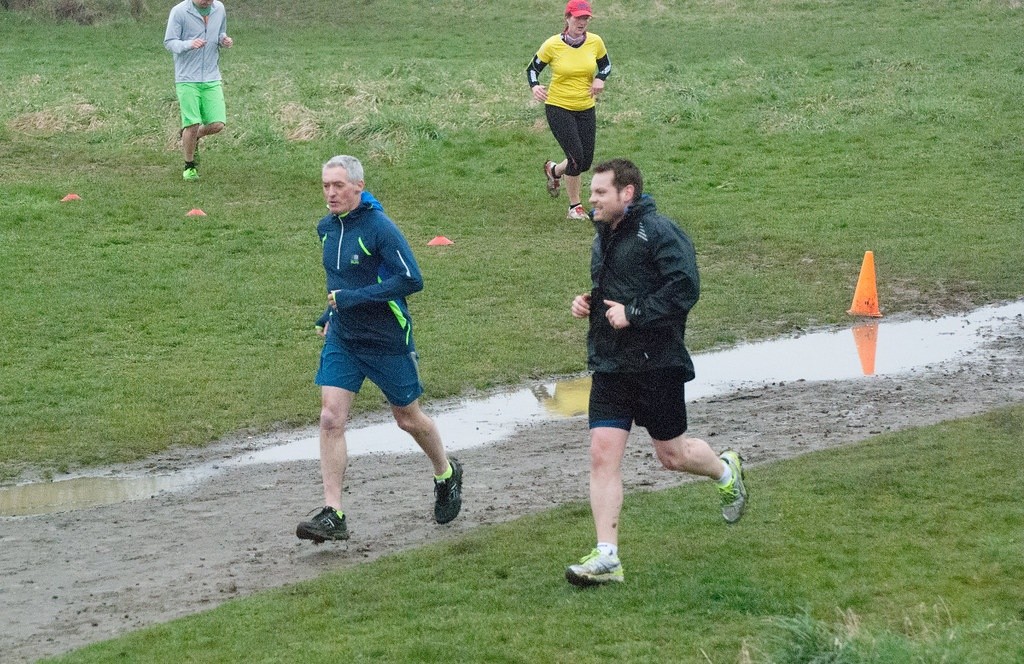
top-left (296, 156), bottom-right (464, 541)
top-left (566, 158), bottom-right (748, 586)
top-left (526, 0), bottom-right (611, 220)
top-left (164, 0), bottom-right (232, 180)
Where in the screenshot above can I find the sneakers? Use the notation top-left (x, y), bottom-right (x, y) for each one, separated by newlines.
top-left (193, 138), bottom-right (202, 166)
top-left (296, 505), bottom-right (350, 541)
top-left (544, 160), bottom-right (562, 198)
top-left (566, 204), bottom-right (591, 221)
top-left (433, 457), bottom-right (463, 525)
top-left (183, 164), bottom-right (200, 182)
top-left (565, 548), bottom-right (624, 587)
top-left (719, 449), bottom-right (749, 524)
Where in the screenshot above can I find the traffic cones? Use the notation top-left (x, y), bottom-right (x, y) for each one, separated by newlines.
top-left (852, 322), bottom-right (879, 375)
top-left (844, 250), bottom-right (884, 318)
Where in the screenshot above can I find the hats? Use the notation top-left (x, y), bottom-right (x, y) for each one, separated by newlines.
top-left (565, 0), bottom-right (593, 18)
